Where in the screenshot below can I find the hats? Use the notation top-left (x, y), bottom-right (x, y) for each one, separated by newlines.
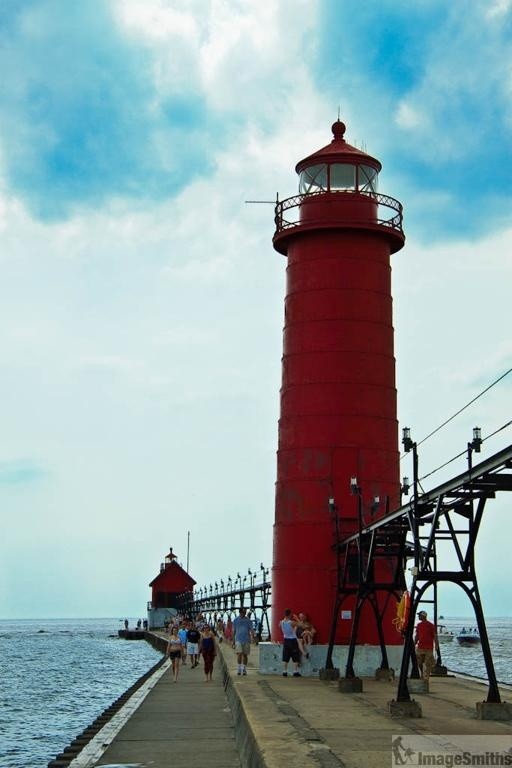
top-left (416, 611), bottom-right (427, 618)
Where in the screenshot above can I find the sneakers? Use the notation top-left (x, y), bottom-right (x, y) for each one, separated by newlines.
top-left (282, 671), bottom-right (302, 679)
top-left (238, 669), bottom-right (247, 675)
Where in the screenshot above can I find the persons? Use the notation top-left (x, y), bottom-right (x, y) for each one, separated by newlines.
top-left (294, 611), bottom-right (316, 659)
top-left (414, 610), bottom-right (438, 680)
top-left (440, 626), bottom-right (478, 635)
top-left (278, 608), bottom-right (303, 677)
top-left (124, 606), bottom-right (264, 683)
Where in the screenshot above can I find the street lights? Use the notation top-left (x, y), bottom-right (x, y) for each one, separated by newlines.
top-left (175, 563), bottom-right (270, 604)
top-left (328, 421), bottom-right (482, 575)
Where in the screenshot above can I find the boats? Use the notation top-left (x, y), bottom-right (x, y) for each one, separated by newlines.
top-left (436, 623), bottom-right (456, 645)
top-left (457, 626), bottom-right (481, 646)
